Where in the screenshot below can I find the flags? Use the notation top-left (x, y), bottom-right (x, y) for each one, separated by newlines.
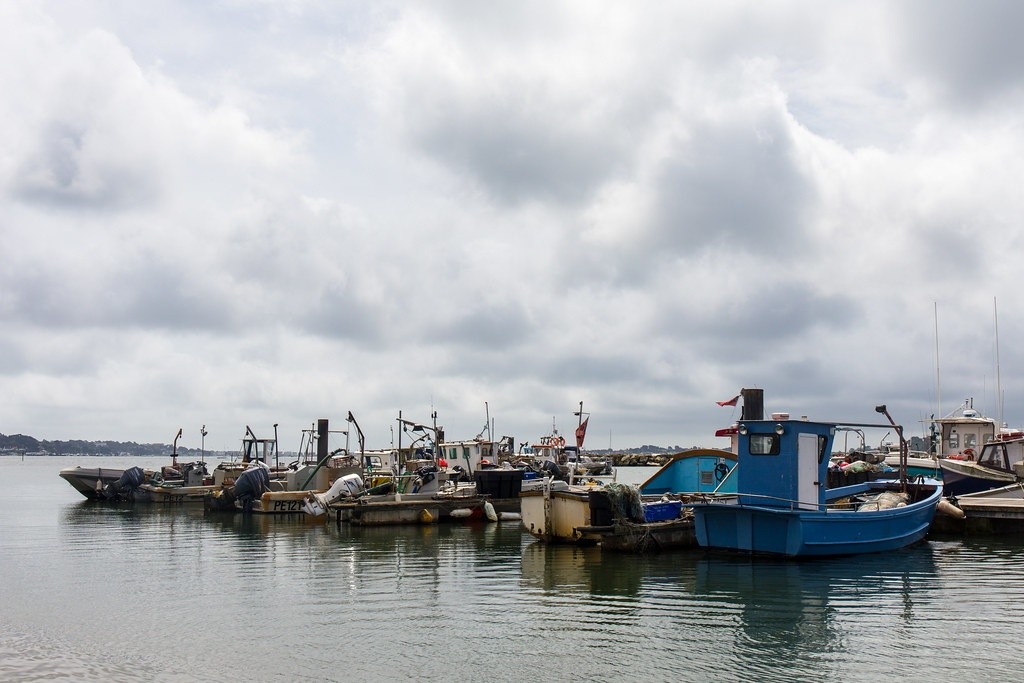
top-left (716, 396), bottom-right (739, 407)
top-left (575, 419), bottom-right (587, 446)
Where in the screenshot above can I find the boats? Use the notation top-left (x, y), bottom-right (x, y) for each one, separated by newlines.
top-left (681, 403), bottom-right (944, 560)
top-left (827, 297), bottom-right (1024, 521)
top-left (516, 388), bottom-right (737, 552)
top-left (59, 410), bottom-right (475, 515)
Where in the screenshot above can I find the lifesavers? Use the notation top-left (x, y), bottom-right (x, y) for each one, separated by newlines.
top-left (550, 438), bottom-right (558, 446)
top-left (949, 456), bottom-right (963, 459)
top-left (963, 449), bottom-right (977, 461)
top-left (557, 437), bottom-right (565, 446)
top-left (482, 460), bottom-right (489, 464)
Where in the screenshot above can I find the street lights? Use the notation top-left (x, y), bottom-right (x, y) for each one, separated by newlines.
top-left (273, 423), bottom-right (279, 472)
